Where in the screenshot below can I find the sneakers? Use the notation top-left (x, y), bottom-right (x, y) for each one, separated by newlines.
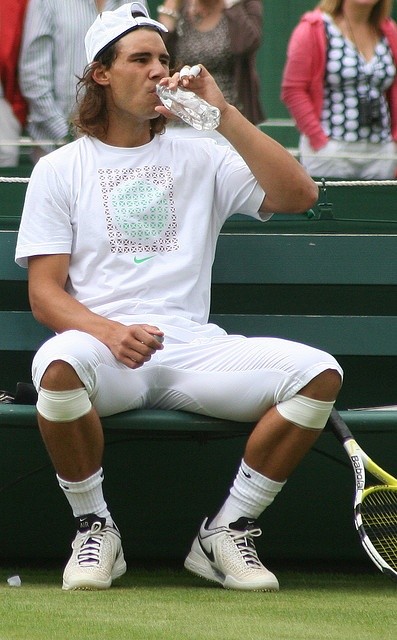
top-left (62, 514), bottom-right (127, 591)
top-left (184, 517), bottom-right (280, 592)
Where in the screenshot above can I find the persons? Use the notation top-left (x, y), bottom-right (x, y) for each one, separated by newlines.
top-left (23, 0), bottom-right (149, 152)
top-left (1, 0), bottom-right (29, 166)
top-left (154, 0), bottom-right (265, 126)
top-left (280, 0), bottom-right (397, 181)
top-left (18, 12), bottom-right (345, 591)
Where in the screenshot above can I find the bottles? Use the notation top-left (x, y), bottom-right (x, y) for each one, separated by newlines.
top-left (155, 83), bottom-right (221, 132)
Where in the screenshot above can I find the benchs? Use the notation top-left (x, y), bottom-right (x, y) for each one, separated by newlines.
top-left (0, 230), bottom-right (395, 432)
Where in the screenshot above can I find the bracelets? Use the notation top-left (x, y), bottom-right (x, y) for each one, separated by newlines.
top-left (157, 6), bottom-right (188, 35)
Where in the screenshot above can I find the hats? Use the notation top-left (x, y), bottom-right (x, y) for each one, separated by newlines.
top-left (85, 2), bottom-right (169, 69)
